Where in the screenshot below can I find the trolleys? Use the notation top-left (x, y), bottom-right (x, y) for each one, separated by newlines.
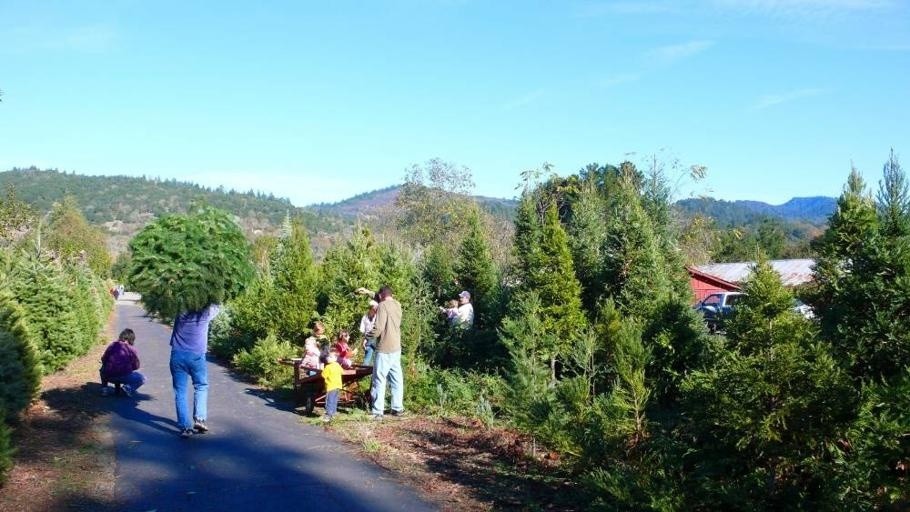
top-left (276, 353), bottom-right (378, 414)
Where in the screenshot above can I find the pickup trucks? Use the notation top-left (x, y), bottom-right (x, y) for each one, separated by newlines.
top-left (690, 287), bottom-right (751, 334)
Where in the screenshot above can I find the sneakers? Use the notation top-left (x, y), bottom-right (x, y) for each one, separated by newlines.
top-left (193, 419), bottom-right (208, 431)
top-left (99, 387), bottom-right (109, 397)
top-left (391, 409), bottom-right (404, 416)
top-left (121, 384), bottom-right (133, 398)
top-left (361, 413), bottom-right (383, 420)
top-left (179, 428), bottom-right (194, 438)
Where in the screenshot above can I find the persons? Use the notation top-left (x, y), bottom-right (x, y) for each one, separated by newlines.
top-left (361, 285), bottom-right (405, 420)
top-left (110, 284), bottom-right (124, 300)
top-left (301, 321), bottom-right (324, 417)
top-left (360, 299), bottom-right (378, 366)
top-left (321, 349), bottom-right (344, 422)
top-left (439, 299), bottom-right (464, 319)
top-left (451, 290), bottom-right (474, 328)
top-left (335, 329), bottom-right (359, 402)
top-left (98, 327), bottom-right (146, 399)
top-left (169, 301), bottom-right (222, 438)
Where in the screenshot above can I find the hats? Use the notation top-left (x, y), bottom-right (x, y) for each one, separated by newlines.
top-left (458, 291), bottom-right (471, 300)
